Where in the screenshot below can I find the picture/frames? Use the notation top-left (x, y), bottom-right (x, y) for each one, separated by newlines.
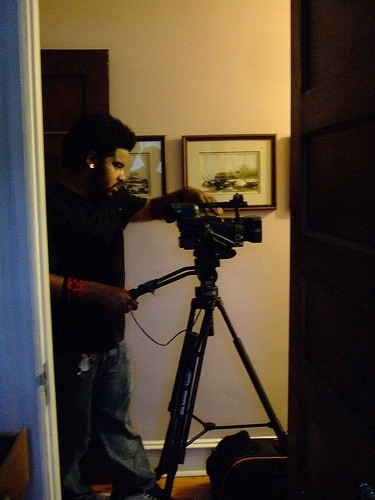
top-left (182, 134), bottom-right (277, 211)
top-left (121, 134), bottom-right (167, 199)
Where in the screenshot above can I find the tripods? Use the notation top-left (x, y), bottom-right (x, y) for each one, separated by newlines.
top-left (127, 259), bottom-right (286, 500)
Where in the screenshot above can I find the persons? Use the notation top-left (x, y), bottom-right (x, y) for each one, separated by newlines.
top-left (48, 114), bottom-right (222, 500)
